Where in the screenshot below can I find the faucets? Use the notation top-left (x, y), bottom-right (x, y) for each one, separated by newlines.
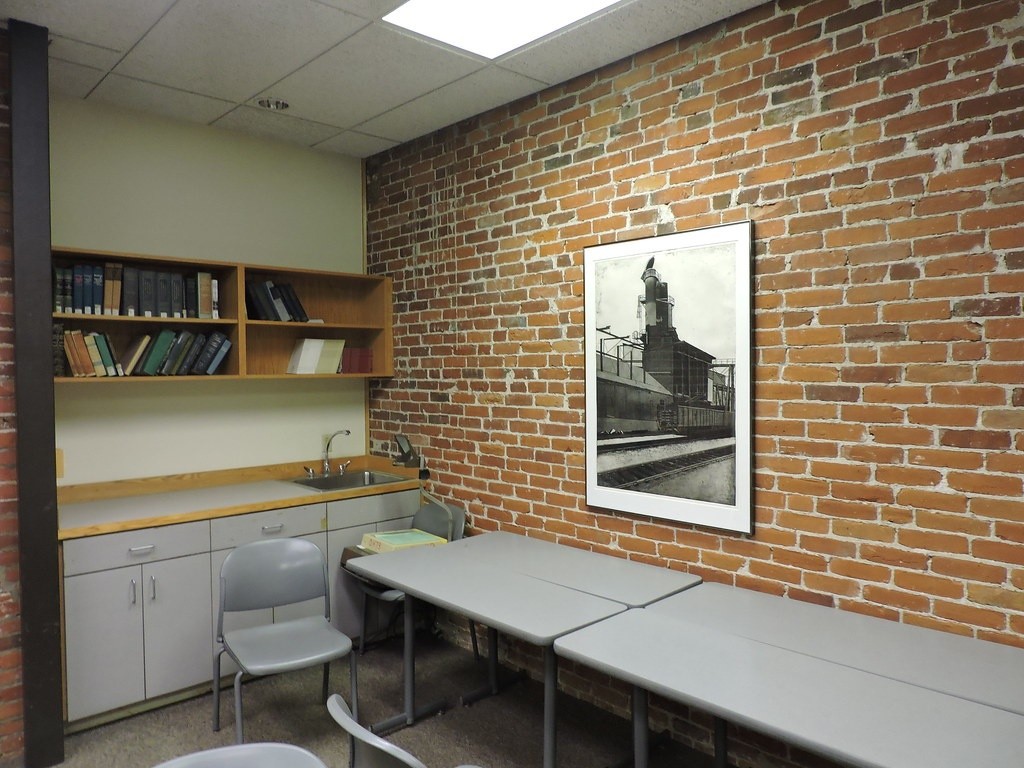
top-left (322, 430), bottom-right (351, 474)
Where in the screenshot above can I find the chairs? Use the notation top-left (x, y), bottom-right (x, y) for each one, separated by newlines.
top-left (149, 742), bottom-right (330, 768)
top-left (325, 693), bottom-right (487, 768)
top-left (211, 538), bottom-right (359, 741)
top-left (353, 503), bottom-right (481, 686)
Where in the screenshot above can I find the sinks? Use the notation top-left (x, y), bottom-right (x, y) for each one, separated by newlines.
top-left (292, 469), bottom-right (407, 492)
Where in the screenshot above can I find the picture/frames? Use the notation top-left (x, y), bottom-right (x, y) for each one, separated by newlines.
top-left (580, 219), bottom-right (759, 536)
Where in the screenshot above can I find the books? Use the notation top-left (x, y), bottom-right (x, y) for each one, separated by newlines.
top-left (53, 263), bottom-right (218, 319)
top-left (53, 323), bottom-right (232, 376)
top-left (245, 282), bottom-right (324, 323)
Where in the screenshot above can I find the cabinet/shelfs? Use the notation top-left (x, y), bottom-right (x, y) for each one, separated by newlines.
top-left (62, 489), bottom-right (423, 737)
top-left (51, 244), bottom-right (396, 382)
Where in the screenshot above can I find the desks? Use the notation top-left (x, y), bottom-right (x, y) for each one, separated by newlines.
top-left (344, 530), bottom-right (1023, 768)
